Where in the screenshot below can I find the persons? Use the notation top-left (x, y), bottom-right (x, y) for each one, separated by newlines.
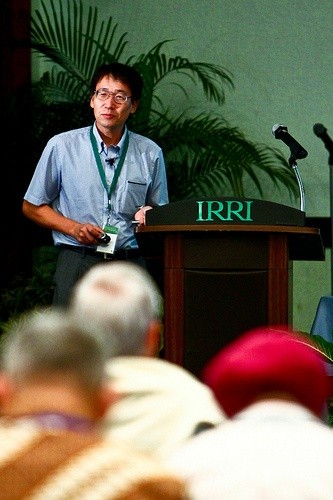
top-left (0, 259), bottom-right (332, 500)
top-left (24, 64), bottom-right (169, 307)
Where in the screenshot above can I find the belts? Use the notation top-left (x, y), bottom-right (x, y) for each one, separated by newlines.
top-left (62, 242), bottom-right (140, 261)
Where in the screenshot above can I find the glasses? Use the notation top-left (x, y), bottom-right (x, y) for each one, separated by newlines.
top-left (94, 89), bottom-right (132, 104)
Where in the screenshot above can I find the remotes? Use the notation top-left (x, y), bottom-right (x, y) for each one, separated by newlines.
top-left (95, 233), bottom-right (111, 244)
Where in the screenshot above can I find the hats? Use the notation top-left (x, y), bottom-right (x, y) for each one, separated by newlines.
top-left (202, 326), bottom-right (333, 419)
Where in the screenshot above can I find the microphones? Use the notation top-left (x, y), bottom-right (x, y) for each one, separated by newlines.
top-left (272, 123), bottom-right (308, 159)
